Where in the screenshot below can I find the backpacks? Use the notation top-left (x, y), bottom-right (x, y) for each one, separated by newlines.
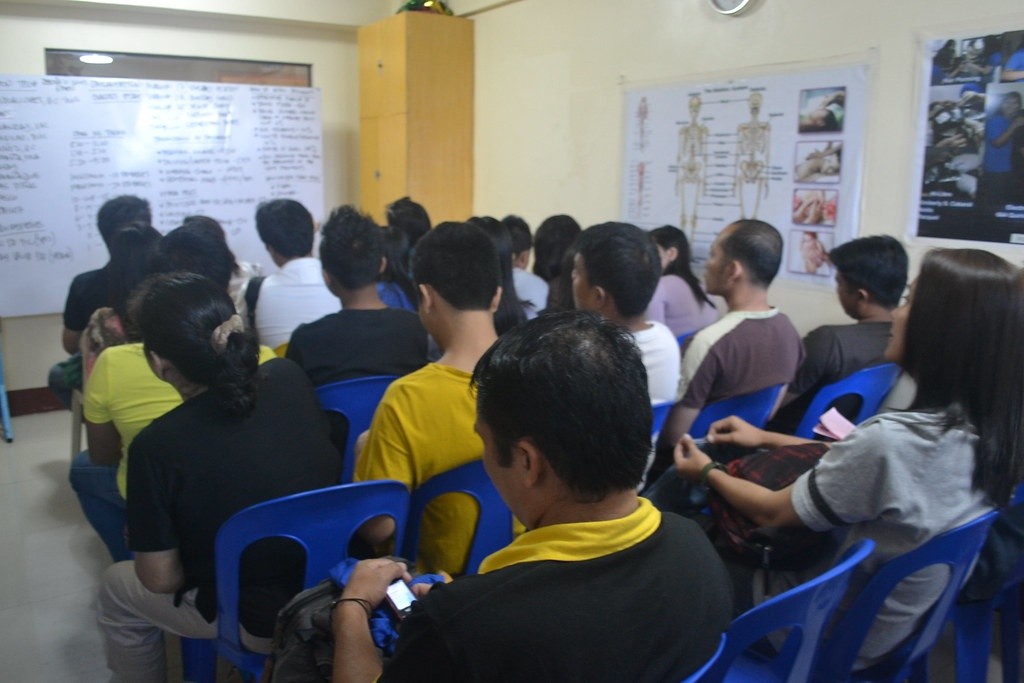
top-left (264, 579), bottom-right (345, 683)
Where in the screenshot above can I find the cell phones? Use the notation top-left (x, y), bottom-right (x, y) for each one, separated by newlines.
top-left (385, 577), bottom-right (419, 622)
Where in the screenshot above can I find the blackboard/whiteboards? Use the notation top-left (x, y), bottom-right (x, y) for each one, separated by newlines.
top-left (0, 73), bottom-right (325, 318)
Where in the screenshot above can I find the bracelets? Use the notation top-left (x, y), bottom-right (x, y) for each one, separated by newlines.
top-left (698, 461), bottom-right (729, 489)
top-left (327, 596), bottom-right (375, 614)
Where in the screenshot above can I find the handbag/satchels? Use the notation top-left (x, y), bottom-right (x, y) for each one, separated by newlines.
top-left (711, 440), bottom-right (849, 567)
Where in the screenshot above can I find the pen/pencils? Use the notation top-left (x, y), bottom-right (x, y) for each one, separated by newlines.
top-left (693, 438), bottom-right (708, 444)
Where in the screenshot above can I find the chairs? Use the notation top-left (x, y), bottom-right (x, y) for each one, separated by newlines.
top-left (182, 363), bottom-right (1024, 682)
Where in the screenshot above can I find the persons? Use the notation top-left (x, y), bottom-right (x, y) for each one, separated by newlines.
top-left (335, 310), bottom-right (730, 683)
top-left (664, 218), bottom-right (804, 447)
top-left (354, 221), bottom-right (526, 580)
top-left (775, 236), bottom-right (908, 433)
top-left (673, 250), bottom-right (1024, 683)
top-left (95, 271), bottom-right (345, 683)
top-left (62, 193), bottom-right (719, 561)
top-left (921, 30), bottom-right (1024, 245)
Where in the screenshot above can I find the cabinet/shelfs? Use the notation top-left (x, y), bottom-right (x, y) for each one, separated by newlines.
top-left (356, 11), bottom-right (475, 226)
